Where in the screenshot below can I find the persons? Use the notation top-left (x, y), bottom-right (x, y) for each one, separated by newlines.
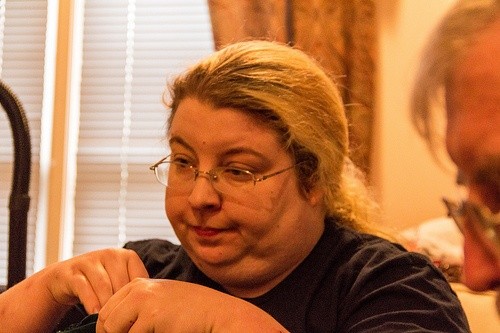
top-left (0, 41), bottom-right (473, 333)
top-left (406, 0), bottom-right (500, 316)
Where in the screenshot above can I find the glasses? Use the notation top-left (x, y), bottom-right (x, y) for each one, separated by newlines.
top-left (150, 153), bottom-right (308, 195)
top-left (442, 196), bottom-right (500, 250)
